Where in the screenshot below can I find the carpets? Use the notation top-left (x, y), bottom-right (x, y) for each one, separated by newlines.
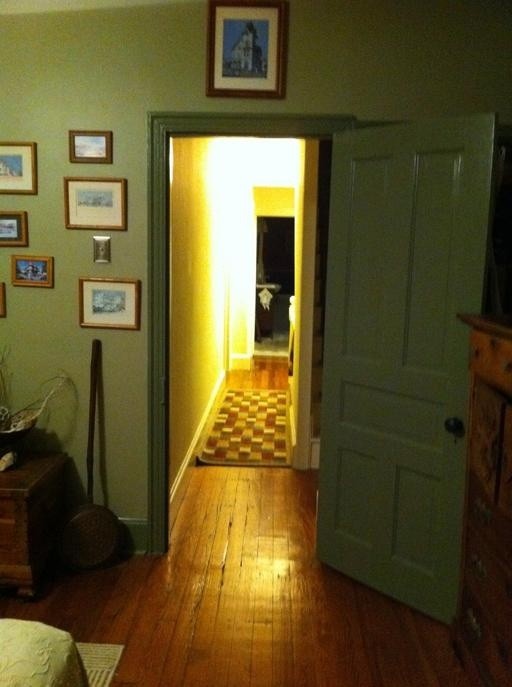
top-left (75, 642), bottom-right (126, 687)
top-left (196, 385), bottom-right (294, 470)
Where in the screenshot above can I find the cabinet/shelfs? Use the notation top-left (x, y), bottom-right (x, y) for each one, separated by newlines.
top-left (453, 305), bottom-right (512, 685)
top-left (1, 449), bottom-right (73, 601)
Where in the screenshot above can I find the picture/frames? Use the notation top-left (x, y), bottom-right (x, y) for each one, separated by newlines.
top-left (0, 131), bottom-right (143, 334)
top-left (202, 0), bottom-right (289, 101)
